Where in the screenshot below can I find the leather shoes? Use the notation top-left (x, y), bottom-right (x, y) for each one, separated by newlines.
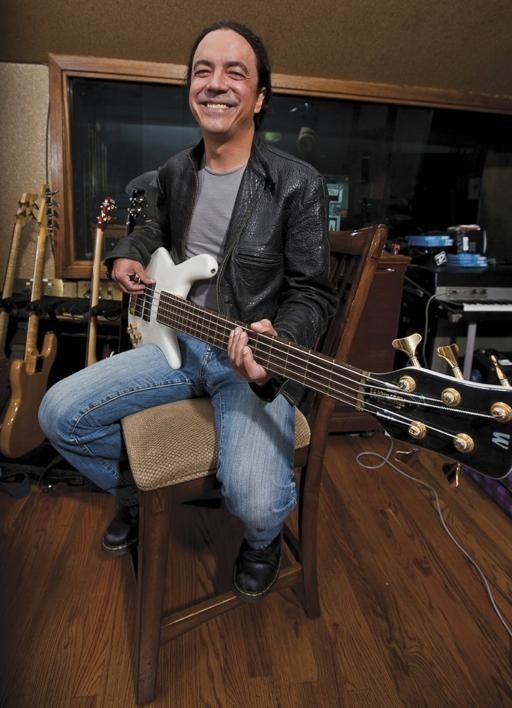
top-left (233, 529), bottom-right (283, 603)
top-left (101, 504), bottom-right (140, 557)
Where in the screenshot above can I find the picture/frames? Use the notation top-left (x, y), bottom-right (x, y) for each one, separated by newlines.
top-left (47, 52), bottom-right (511, 281)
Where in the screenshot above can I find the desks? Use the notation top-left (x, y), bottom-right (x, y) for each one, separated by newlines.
top-left (328, 252), bottom-right (411, 435)
top-left (394, 277), bottom-right (512, 380)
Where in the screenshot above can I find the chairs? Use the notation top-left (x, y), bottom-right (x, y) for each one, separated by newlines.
top-left (120, 223), bottom-right (389, 707)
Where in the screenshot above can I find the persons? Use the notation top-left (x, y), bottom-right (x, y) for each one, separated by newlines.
top-left (38, 21), bottom-right (336, 600)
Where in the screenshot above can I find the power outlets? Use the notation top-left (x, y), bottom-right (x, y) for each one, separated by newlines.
top-left (78, 282), bottom-right (91, 297)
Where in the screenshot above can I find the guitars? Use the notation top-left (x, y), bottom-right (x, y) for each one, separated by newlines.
top-left (0, 192), bottom-right (39, 428)
top-left (127, 247), bottom-right (512, 479)
top-left (1, 184), bottom-right (60, 459)
